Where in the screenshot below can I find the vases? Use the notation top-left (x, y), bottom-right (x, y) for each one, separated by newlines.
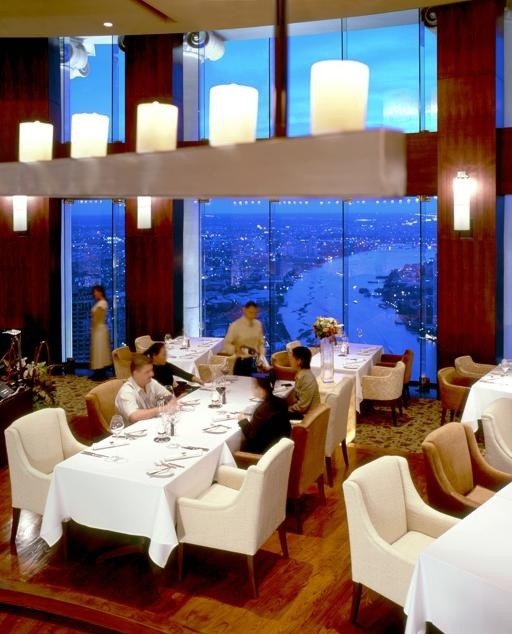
top-left (321, 337), bottom-right (336, 383)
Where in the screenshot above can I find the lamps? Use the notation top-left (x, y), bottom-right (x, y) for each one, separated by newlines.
top-left (453, 178), bottom-right (470, 230)
top-left (18, 121), bottom-right (53, 163)
top-left (208, 83), bottom-right (258, 147)
top-left (69, 113), bottom-right (108, 159)
top-left (135, 102), bottom-right (177, 152)
top-left (12, 196), bottom-right (28, 232)
top-left (137, 194), bottom-right (151, 230)
top-left (310, 60), bottom-right (370, 135)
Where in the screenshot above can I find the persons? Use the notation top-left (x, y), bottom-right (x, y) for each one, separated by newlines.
top-left (236, 370), bottom-right (292, 454)
top-left (222, 300), bottom-right (264, 376)
top-left (288, 345), bottom-right (321, 420)
top-left (142, 341), bottom-right (204, 395)
top-left (88, 285), bottom-right (114, 383)
top-left (114, 353), bottom-right (177, 424)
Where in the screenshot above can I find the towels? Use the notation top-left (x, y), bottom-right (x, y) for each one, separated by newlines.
top-left (345, 357), bottom-right (366, 366)
top-left (173, 404), bottom-right (194, 413)
top-left (203, 381), bottom-right (234, 388)
top-left (164, 448), bottom-right (203, 462)
top-left (274, 386), bottom-right (287, 393)
top-left (93, 438), bottom-right (131, 452)
top-left (213, 415), bottom-right (239, 421)
top-left (361, 346), bottom-right (379, 352)
top-left (203, 339), bottom-right (220, 344)
top-left (183, 349), bottom-right (206, 356)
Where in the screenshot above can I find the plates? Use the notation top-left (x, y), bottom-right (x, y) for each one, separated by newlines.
top-left (343, 365), bottom-right (360, 369)
top-left (124, 430), bottom-right (148, 437)
top-left (358, 353), bottom-right (371, 356)
top-left (148, 469), bottom-right (176, 477)
top-left (205, 428), bottom-right (228, 434)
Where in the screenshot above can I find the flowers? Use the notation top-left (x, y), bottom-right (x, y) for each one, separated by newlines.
top-left (312, 317), bottom-right (339, 345)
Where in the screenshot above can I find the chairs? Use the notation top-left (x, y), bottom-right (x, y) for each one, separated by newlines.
top-left (362, 362), bottom-right (405, 427)
top-left (285, 341), bottom-right (319, 356)
top-left (453, 355), bottom-right (497, 378)
top-left (377, 349), bottom-right (413, 409)
top-left (135, 335), bottom-right (162, 353)
top-left (271, 350), bottom-right (296, 380)
top-left (234, 404), bottom-right (331, 558)
top-left (481, 398), bottom-right (511, 471)
top-left (422, 422), bottom-right (512, 509)
top-left (437, 366), bottom-right (486, 425)
top-left (199, 354), bottom-right (232, 383)
top-left (112, 346), bottom-right (132, 378)
top-left (288, 377), bottom-right (356, 488)
top-left (176, 438), bottom-right (296, 599)
top-left (342, 454), bottom-right (463, 625)
top-left (4, 407), bottom-right (89, 558)
top-left (85, 379), bottom-right (126, 433)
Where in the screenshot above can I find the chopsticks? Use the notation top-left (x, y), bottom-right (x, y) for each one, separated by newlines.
top-left (81, 450), bottom-right (108, 459)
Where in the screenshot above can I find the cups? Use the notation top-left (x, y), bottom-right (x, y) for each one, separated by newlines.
top-left (110, 415), bottom-right (125, 446)
top-left (162, 385), bottom-right (174, 405)
top-left (215, 377), bottom-right (227, 406)
top-left (164, 333), bottom-right (172, 349)
top-left (500, 359), bottom-right (510, 377)
top-left (356, 327), bottom-right (364, 346)
